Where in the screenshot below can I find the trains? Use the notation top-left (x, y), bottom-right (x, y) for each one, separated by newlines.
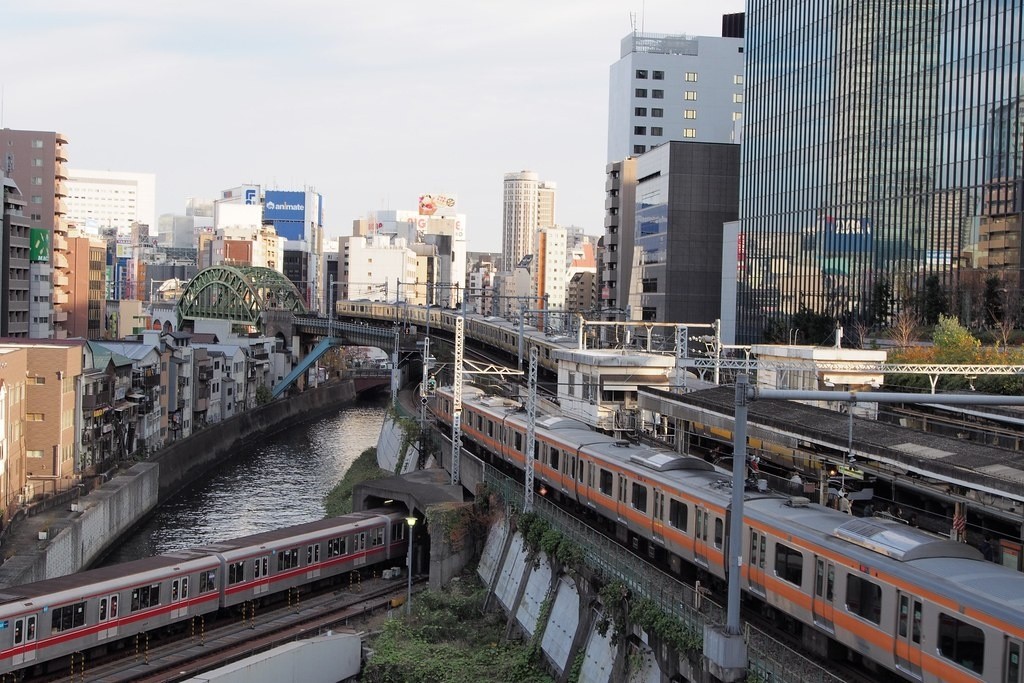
top-left (1, 506), bottom-right (421, 682)
top-left (436, 384), bottom-right (1024, 683)
top-left (335, 299), bottom-right (589, 375)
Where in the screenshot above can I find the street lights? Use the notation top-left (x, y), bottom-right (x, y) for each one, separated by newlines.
top-left (405, 517), bottom-right (418, 615)
